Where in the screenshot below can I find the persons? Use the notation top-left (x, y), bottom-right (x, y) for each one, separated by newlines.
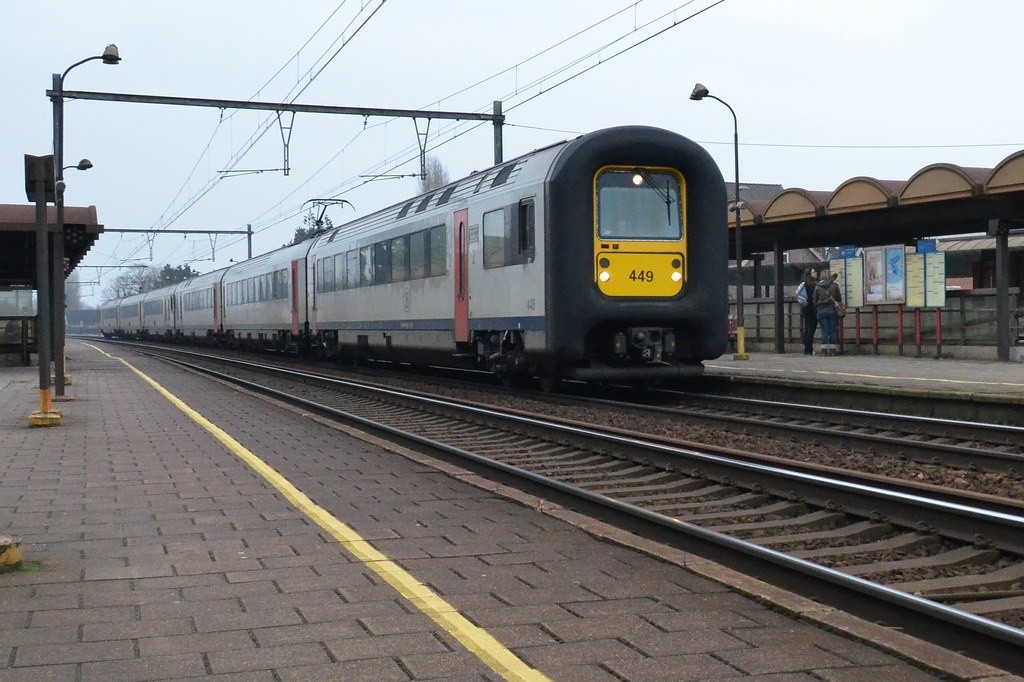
top-left (814, 269), bottom-right (842, 356)
top-left (801, 267), bottom-right (840, 356)
top-left (617, 218), bottom-right (626, 232)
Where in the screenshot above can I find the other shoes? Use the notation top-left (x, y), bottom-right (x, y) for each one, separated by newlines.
top-left (805, 351), bottom-right (820, 357)
top-left (829, 348), bottom-right (841, 356)
top-left (821, 348), bottom-right (830, 356)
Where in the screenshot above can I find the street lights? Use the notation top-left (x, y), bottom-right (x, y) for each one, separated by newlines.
top-left (690, 84), bottom-right (749, 361)
top-left (62, 159), bottom-right (92, 174)
top-left (50, 44), bottom-right (122, 401)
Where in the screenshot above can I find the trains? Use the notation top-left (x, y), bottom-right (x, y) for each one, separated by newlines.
top-left (100, 124), bottom-right (730, 388)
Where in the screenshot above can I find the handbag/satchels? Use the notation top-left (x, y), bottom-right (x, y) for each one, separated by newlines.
top-left (834, 301), bottom-right (846, 318)
